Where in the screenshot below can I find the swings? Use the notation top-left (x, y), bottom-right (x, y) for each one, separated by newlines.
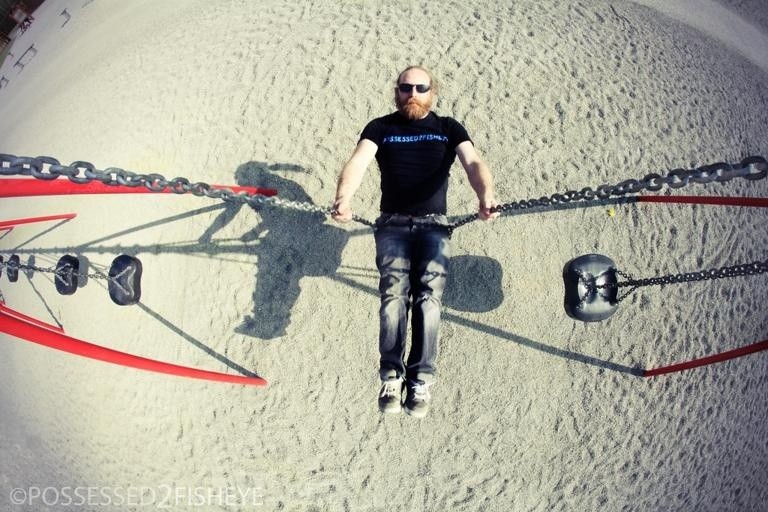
top-left (0, 152), bottom-right (768, 320)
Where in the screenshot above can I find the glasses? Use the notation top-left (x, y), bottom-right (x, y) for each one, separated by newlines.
top-left (398, 83), bottom-right (431, 93)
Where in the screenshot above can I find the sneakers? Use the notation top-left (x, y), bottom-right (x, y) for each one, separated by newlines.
top-left (377, 367), bottom-right (433, 419)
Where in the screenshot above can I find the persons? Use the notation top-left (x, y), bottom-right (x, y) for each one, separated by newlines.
top-left (330, 67), bottom-right (499, 415)
